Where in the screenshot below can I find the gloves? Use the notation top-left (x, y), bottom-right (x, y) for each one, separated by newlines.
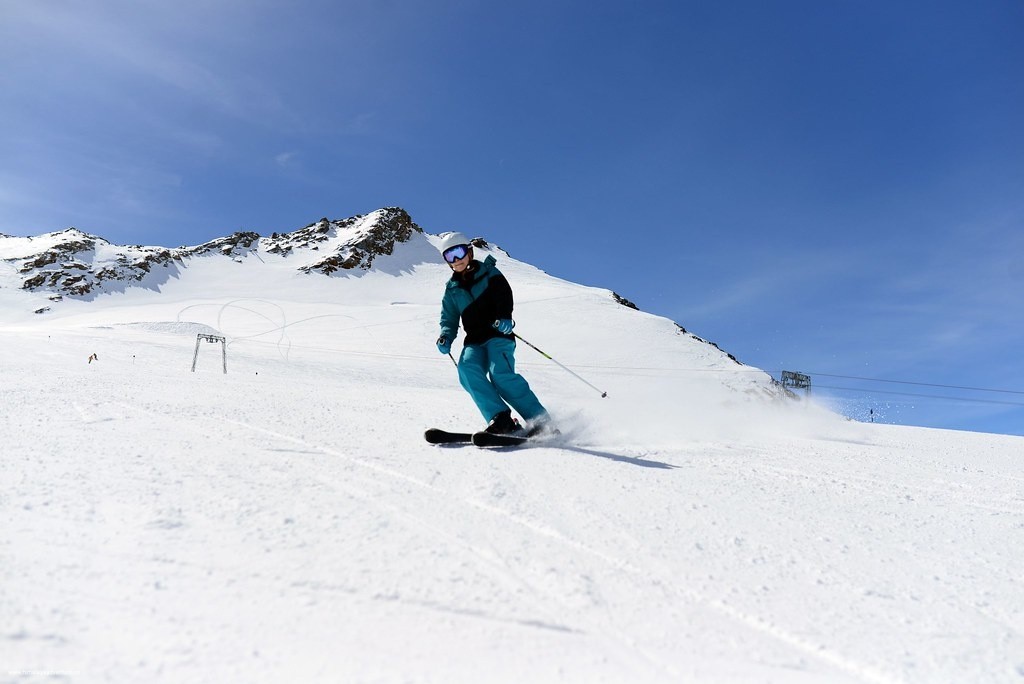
top-left (492, 319), bottom-right (512, 335)
top-left (437, 337), bottom-right (451, 354)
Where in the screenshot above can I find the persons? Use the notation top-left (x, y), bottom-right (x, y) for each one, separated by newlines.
top-left (436, 232), bottom-right (560, 436)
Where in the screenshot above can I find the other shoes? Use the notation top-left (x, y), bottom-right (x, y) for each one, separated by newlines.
top-left (516, 420), bottom-right (561, 438)
top-left (485, 412), bottom-right (515, 434)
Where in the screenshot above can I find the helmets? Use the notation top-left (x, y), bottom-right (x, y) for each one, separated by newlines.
top-left (439, 232), bottom-right (472, 268)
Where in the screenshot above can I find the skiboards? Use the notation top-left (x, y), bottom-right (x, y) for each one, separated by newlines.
top-left (424, 428), bottom-right (562, 450)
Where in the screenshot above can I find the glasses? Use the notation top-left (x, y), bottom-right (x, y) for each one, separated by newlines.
top-left (443, 245), bottom-right (468, 263)
